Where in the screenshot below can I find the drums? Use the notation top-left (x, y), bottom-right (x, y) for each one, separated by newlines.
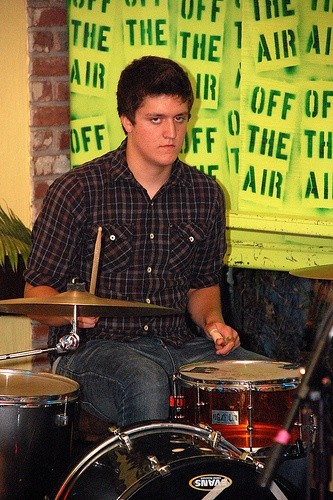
top-left (0, 368), bottom-right (81, 500)
top-left (55, 418), bottom-right (287, 500)
top-left (172, 359), bottom-right (319, 464)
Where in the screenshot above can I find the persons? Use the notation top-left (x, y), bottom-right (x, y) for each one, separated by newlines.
top-left (24, 55), bottom-right (283, 500)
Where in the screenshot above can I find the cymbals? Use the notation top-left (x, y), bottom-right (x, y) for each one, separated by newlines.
top-left (0, 283), bottom-right (186, 317)
top-left (288, 263), bottom-right (333, 281)
